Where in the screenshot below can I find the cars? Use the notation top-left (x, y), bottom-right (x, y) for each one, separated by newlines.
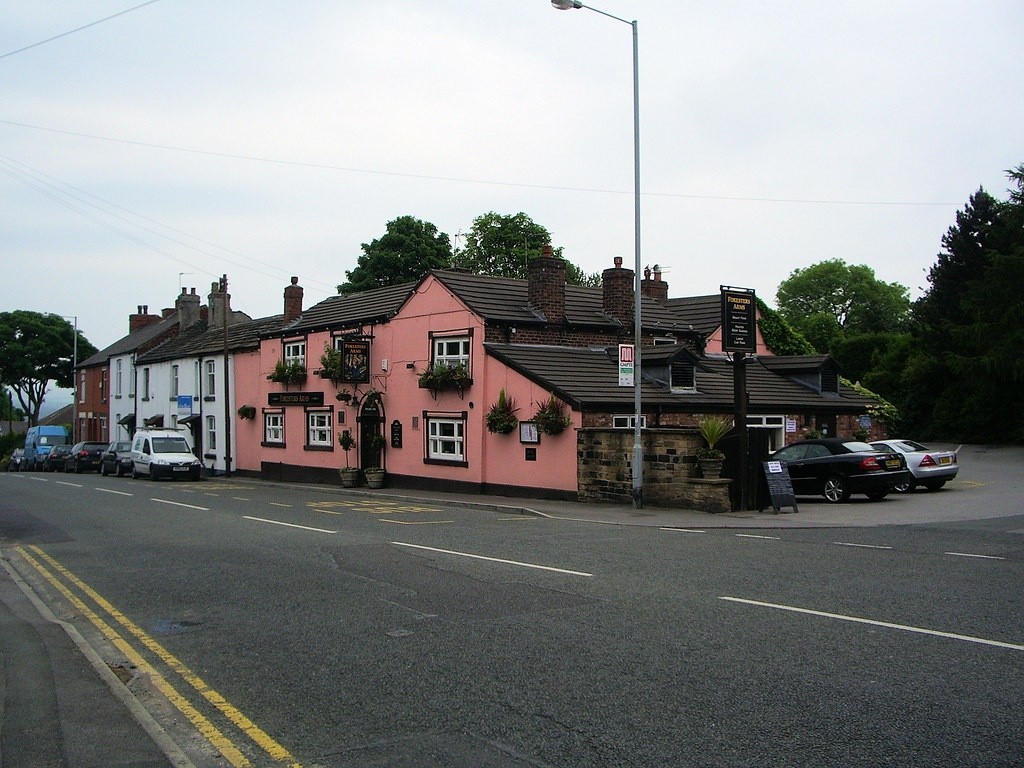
top-left (43, 444), bottom-right (73, 472)
top-left (9, 448), bottom-right (25, 472)
top-left (97, 441), bottom-right (133, 478)
top-left (766, 437), bottom-right (911, 504)
top-left (867, 438), bottom-right (960, 494)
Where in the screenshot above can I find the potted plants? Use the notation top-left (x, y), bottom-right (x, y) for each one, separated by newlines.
top-left (237, 404), bottom-right (256, 420)
top-left (696, 414), bottom-right (735, 479)
top-left (414, 361), bottom-right (473, 389)
top-left (853, 432), bottom-right (869, 442)
top-left (530, 390), bottom-right (571, 437)
top-left (367, 387), bottom-right (382, 409)
top-left (363, 434), bottom-right (386, 488)
top-left (486, 388), bottom-right (521, 435)
top-left (319, 344), bottom-right (341, 387)
top-left (336, 390), bottom-right (352, 407)
top-left (270, 356), bottom-right (307, 389)
top-left (338, 433), bottom-right (359, 487)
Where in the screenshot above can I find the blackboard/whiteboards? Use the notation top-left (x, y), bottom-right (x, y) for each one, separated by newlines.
top-left (761, 460), bottom-right (798, 509)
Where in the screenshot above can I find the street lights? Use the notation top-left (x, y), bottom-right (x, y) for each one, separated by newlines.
top-left (63, 315), bottom-right (77, 447)
top-left (549, 0), bottom-right (644, 511)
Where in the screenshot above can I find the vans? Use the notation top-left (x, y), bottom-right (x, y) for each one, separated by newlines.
top-left (25, 424), bottom-right (68, 472)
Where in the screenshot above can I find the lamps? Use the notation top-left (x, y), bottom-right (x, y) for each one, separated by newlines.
top-left (350, 389), bottom-right (365, 410)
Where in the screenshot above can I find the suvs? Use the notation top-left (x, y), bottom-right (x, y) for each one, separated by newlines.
top-left (63, 440), bottom-right (110, 474)
top-left (130, 427), bottom-right (201, 482)
